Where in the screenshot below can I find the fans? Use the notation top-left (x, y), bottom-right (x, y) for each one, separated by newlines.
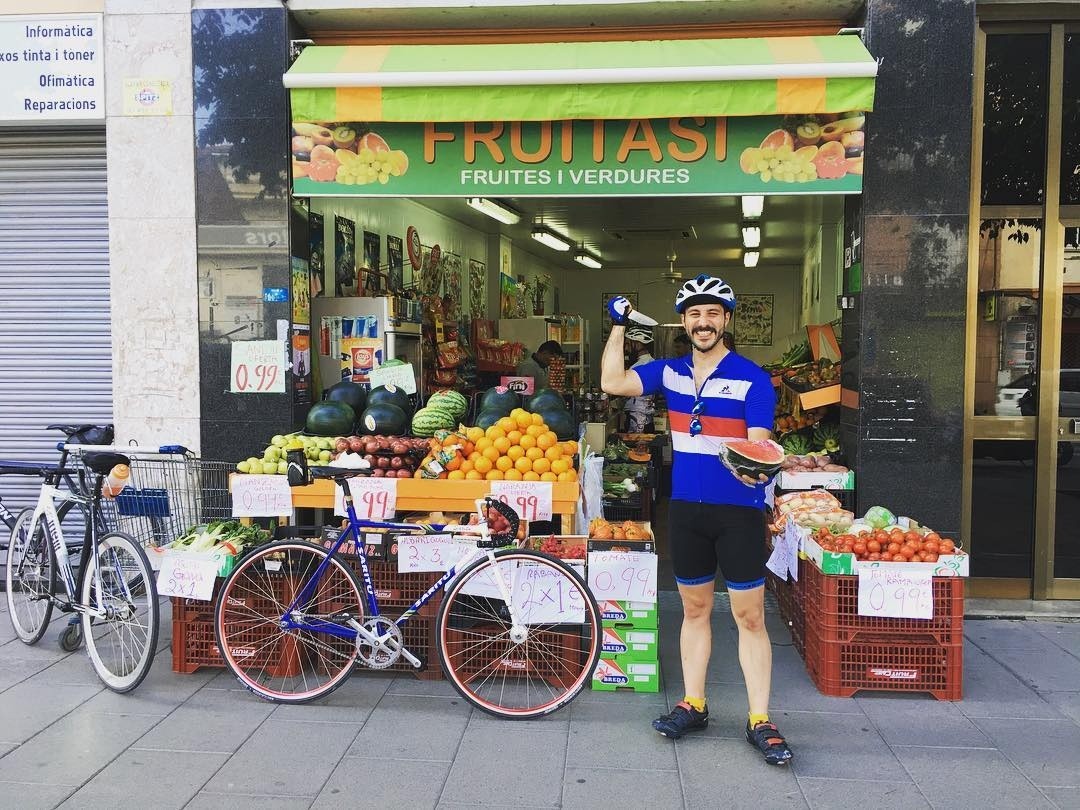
top-left (638, 241), bottom-right (697, 288)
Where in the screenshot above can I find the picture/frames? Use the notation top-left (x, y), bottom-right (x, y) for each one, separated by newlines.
top-left (601, 294), bottom-right (639, 343)
top-left (734, 294), bottom-right (774, 345)
top-left (500, 272), bottom-right (517, 320)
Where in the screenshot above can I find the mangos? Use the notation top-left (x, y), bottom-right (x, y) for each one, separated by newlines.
top-left (292, 123), bottom-right (332, 146)
top-left (819, 116), bottom-right (866, 140)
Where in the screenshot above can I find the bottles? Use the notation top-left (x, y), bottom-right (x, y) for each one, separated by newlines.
top-left (551, 311), bottom-right (582, 342)
top-left (102, 463), bottom-right (129, 500)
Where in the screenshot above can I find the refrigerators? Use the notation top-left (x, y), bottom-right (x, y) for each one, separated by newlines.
top-left (310, 297), bottom-right (423, 409)
top-left (498, 317), bottom-right (560, 355)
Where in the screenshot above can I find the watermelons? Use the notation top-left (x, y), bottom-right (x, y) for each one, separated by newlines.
top-left (304, 383), bottom-right (410, 437)
top-left (412, 406), bottom-right (456, 437)
top-left (427, 390), bottom-right (468, 423)
top-left (780, 431), bottom-right (814, 455)
top-left (722, 439), bottom-right (784, 480)
top-left (475, 385), bottom-right (576, 440)
top-left (812, 422), bottom-right (840, 450)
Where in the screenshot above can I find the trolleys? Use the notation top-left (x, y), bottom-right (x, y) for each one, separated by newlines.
top-left (57, 442), bottom-right (210, 653)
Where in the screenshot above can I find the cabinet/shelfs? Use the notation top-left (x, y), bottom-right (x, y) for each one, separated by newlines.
top-left (762, 357), bottom-right (842, 458)
top-left (422, 317), bottom-right (527, 423)
top-left (562, 319), bottom-right (589, 385)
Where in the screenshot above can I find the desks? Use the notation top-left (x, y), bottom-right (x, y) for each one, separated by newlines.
top-left (586, 410), bottom-right (625, 453)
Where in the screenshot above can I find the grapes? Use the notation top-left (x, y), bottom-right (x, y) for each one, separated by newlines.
top-left (335, 149), bottom-right (400, 185)
top-left (759, 144), bottom-right (818, 182)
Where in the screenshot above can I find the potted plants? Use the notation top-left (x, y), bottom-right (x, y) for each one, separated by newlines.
top-left (526, 274), bottom-right (553, 315)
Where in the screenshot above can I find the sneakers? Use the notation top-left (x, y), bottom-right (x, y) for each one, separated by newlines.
top-left (652, 699), bottom-right (709, 738)
top-left (746, 718), bottom-right (794, 765)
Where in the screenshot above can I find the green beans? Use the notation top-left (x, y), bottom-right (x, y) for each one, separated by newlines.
top-left (811, 522), bottom-right (849, 534)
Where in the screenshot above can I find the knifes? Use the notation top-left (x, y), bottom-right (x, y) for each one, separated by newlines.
top-left (625, 307), bottom-right (659, 326)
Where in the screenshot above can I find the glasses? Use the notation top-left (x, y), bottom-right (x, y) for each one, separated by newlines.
top-left (689, 400), bottom-right (705, 437)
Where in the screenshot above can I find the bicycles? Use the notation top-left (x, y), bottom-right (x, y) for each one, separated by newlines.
top-left (0, 423), bottom-right (160, 694)
top-left (214, 463), bottom-right (610, 721)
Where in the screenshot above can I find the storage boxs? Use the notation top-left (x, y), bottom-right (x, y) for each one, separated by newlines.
top-left (168, 546), bottom-right (661, 697)
top-left (766, 470), bottom-right (963, 702)
top-left (602, 411), bottom-right (674, 522)
top-left (302, 381), bottom-right (578, 441)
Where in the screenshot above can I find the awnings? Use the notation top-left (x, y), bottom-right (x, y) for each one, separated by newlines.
top-left (282, 36), bottom-right (879, 124)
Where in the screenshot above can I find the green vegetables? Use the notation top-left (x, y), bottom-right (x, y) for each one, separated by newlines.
top-left (602, 438), bottom-right (647, 497)
top-left (172, 520), bottom-right (271, 555)
top-left (781, 343), bottom-right (808, 368)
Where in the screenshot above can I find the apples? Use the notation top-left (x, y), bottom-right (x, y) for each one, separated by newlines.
top-left (778, 406), bottom-right (827, 432)
top-left (460, 506), bottom-right (526, 542)
top-left (813, 527), bottom-right (956, 562)
top-left (820, 130), bottom-right (865, 159)
top-left (292, 136), bottom-right (336, 164)
top-left (237, 434), bottom-right (434, 478)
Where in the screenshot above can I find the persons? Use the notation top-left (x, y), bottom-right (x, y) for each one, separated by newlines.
top-left (600, 273), bottom-right (794, 766)
top-left (673, 333), bottom-right (691, 358)
top-left (624, 325), bottom-right (658, 435)
top-left (517, 341), bottom-right (562, 390)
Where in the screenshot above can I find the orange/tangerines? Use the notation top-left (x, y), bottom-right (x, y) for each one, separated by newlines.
top-left (439, 408), bottom-right (579, 481)
top-left (759, 129), bottom-right (818, 163)
top-left (336, 133), bottom-right (390, 165)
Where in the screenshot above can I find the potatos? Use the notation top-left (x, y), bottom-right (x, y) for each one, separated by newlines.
top-left (781, 455), bottom-right (848, 471)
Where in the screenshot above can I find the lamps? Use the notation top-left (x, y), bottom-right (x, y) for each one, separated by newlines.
top-left (463, 198), bottom-right (604, 271)
top-left (740, 196), bottom-right (768, 269)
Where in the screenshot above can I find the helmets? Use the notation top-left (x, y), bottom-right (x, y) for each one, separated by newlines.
top-left (674, 274), bottom-right (737, 311)
top-left (625, 325), bottom-right (655, 344)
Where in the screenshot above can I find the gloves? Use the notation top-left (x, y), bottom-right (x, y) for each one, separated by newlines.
top-left (607, 295), bottom-right (633, 325)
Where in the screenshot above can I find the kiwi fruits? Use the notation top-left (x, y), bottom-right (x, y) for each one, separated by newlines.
top-left (797, 122), bottom-right (821, 146)
top-left (332, 126), bottom-right (356, 148)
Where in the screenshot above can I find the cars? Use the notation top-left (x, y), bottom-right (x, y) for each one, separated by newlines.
top-left (973, 358), bottom-right (1080, 467)
